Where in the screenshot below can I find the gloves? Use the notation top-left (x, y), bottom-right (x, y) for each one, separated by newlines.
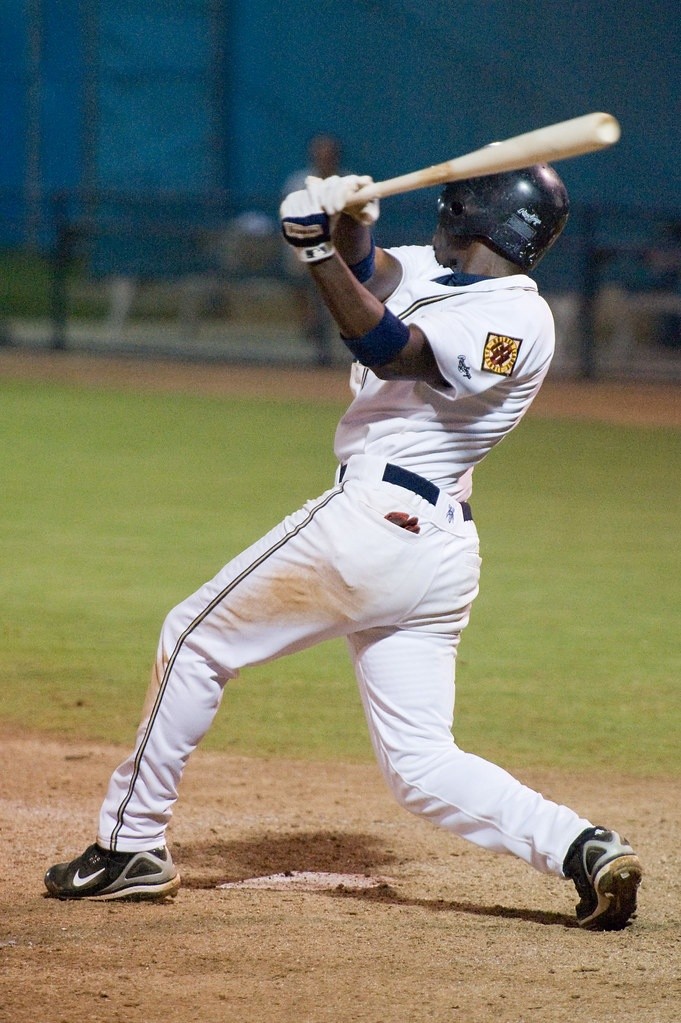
top-left (280, 175), bottom-right (380, 265)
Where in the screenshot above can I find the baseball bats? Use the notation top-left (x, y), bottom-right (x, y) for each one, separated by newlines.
top-left (336, 112), bottom-right (620, 210)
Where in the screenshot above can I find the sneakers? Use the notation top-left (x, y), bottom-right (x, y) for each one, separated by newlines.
top-left (44, 842), bottom-right (181, 901)
top-left (574, 826), bottom-right (643, 931)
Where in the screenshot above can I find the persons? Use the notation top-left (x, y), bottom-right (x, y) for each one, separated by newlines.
top-left (280, 135), bottom-right (342, 366)
top-left (44, 162), bottom-right (643, 930)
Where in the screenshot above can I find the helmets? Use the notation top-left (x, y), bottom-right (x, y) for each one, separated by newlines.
top-left (436, 141), bottom-right (571, 271)
